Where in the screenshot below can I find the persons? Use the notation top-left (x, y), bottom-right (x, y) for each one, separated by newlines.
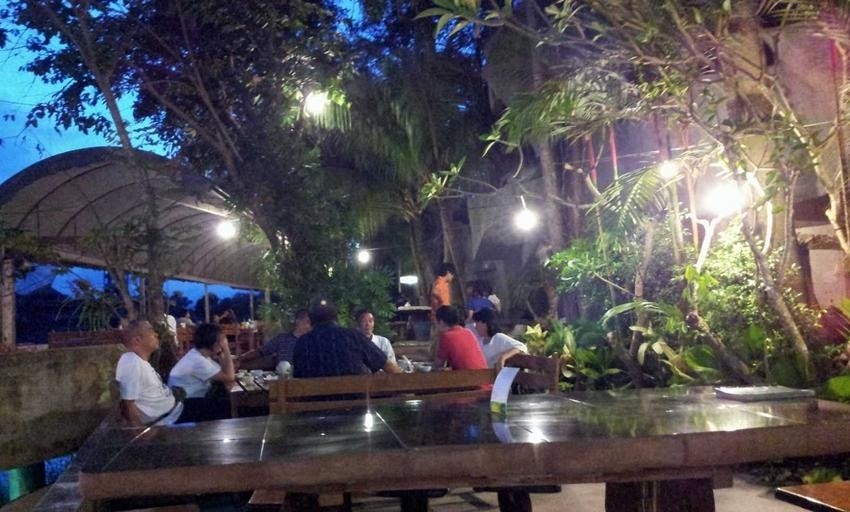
top-left (481, 284), bottom-right (501, 311)
top-left (425, 304), bottom-right (494, 369)
top-left (470, 307), bottom-right (530, 372)
top-left (292, 305), bottom-right (408, 374)
top-left (165, 323), bottom-right (236, 397)
top-left (427, 262), bottom-right (454, 308)
top-left (237, 308), bottom-right (314, 370)
top-left (112, 317), bottom-right (230, 432)
top-left (463, 281), bottom-right (499, 321)
top-left (161, 309), bottom-right (238, 347)
top-left (356, 308), bottom-right (403, 374)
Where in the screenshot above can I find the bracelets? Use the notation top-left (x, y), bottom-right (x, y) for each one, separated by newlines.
top-left (238, 355), bottom-right (249, 362)
top-left (215, 350), bottom-right (223, 355)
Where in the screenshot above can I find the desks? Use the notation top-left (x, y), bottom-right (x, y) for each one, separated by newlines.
top-left (78, 383), bottom-right (850, 511)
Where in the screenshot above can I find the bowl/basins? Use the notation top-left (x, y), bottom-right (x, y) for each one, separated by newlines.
top-left (398, 358), bottom-right (411, 371)
top-left (417, 365), bottom-right (433, 374)
top-left (231, 368), bottom-right (265, 384)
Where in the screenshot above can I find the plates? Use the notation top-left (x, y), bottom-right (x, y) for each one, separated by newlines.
top-left (710, 384), bottom-right (817, 404)
